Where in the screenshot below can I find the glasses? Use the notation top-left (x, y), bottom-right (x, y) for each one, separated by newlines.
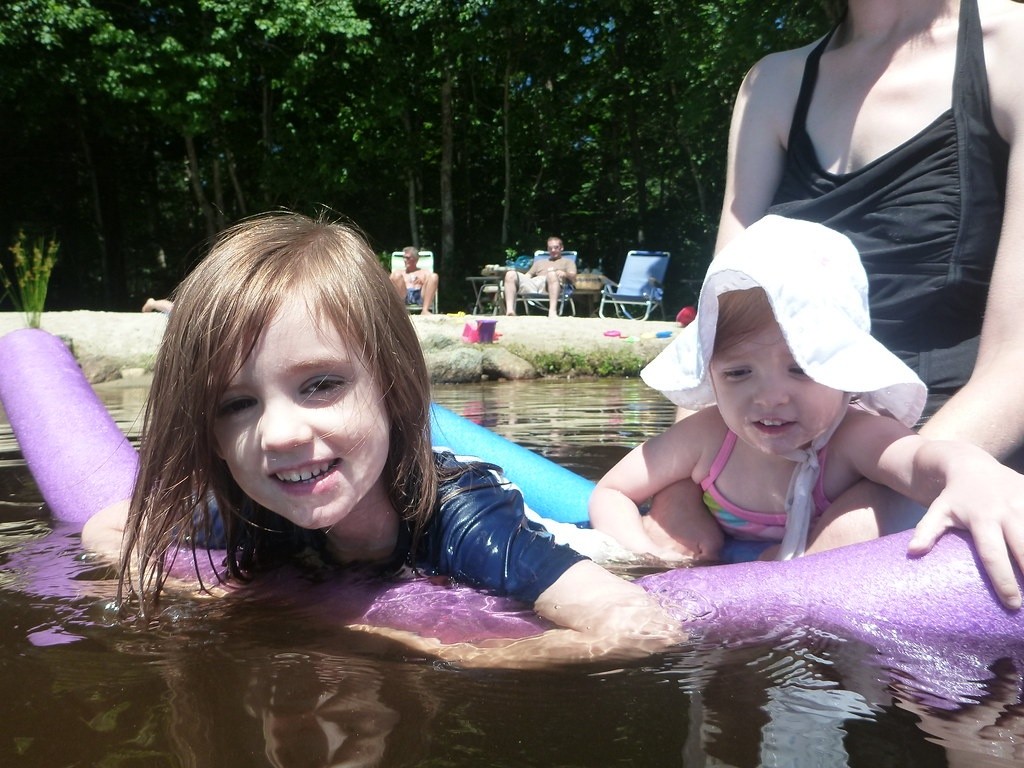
top-left (548, 246), bottom-right (559, 249)
top-left (402, 256), bottom-right (416, 260)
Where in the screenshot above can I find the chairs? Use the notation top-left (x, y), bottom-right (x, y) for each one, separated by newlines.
top-left (391, 251), bottom-right (439, 314)
top-left (516, 251), bottom-right (578, 317)
top-left (599, 250), bottom-right (671, 322)
top-left (465, 256), bottom-right (534, 316)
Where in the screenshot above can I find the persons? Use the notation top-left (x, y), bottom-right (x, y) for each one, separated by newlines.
top-left (643, 0), bottom-right (1023, 564)
top-left (505, 236), bottom-right (577, 318)
top-left (389, 246), bottom-right (440, 316)
top-left (81, 208), bottom-right (640, 632)
top-left (588, 211), bottom-right (1024, 614)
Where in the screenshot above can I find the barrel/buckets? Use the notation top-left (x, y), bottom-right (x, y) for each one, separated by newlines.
top-left (477, 320), bottom-right (497, 343)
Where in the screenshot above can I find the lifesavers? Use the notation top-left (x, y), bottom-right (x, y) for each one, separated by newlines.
top-left (1, 326), bottom-right (1022, 671)
top-left (424, 395), bottom-right (770, 564)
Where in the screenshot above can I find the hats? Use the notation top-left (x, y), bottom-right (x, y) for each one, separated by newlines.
top-left (638, 213), bottom-right (928, 430)
